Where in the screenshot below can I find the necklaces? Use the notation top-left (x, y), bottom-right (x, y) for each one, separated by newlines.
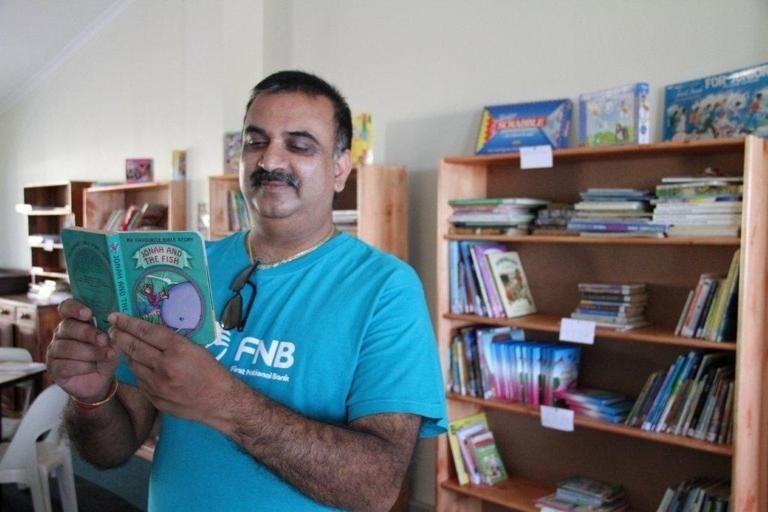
top-left (248, 220), bottom-right (336, 270)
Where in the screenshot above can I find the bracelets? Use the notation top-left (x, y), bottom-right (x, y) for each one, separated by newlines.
top-left (71, 380), bottom-right (118, 407)
top-left (75, 377), bottom-right (114, 411)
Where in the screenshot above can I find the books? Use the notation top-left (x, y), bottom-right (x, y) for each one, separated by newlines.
top-left (100, 201), bottom-right (167, 232)
top-left (58, 225), bottom-right (217, 347)
top-left (332, 209), bottom-right (358, 224)
top-left (228, 188), bottom-right (251, 232)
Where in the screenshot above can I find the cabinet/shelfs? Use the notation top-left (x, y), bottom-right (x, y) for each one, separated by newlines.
top-left (23, 179), bottom-right (90, 289)
top-left (209, 168), bottom-right (408, 286)
top-left (426, 134), bottom-right (766, 512)
top-left (2, 271), bottom-right (75, 361)
top-left (82, 180), bottom-right (182, 230)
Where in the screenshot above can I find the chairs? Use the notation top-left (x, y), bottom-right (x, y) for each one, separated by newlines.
top-left (1, 384), bottom-right (83, 510)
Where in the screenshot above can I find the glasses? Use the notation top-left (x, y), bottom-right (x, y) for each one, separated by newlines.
top-left (220, 258), bottom-right (263, 332)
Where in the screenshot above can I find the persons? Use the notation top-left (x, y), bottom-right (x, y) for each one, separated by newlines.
top-left (43, 69), bottom-right (448, 512)
